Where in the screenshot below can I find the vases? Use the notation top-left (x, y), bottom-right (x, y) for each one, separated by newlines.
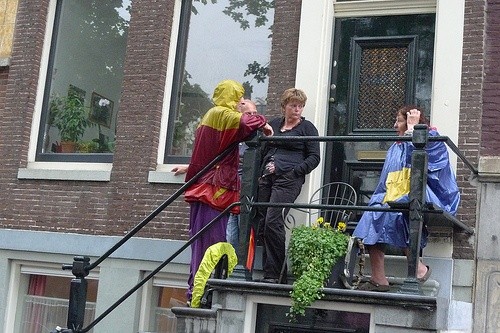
top-left (324, 239), bottom-right (363, 290)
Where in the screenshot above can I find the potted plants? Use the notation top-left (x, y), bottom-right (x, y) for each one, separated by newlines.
top-left (50, 97), bottom-right (83, 152)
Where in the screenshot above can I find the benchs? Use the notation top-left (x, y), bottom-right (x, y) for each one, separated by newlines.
top-left (390, 199), bottom-right (473, 237)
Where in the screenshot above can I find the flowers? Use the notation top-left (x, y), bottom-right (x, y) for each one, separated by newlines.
top-left (286, 217), bottom-right (346, 321)
top-left (96, 99), bottom-right (110, 151)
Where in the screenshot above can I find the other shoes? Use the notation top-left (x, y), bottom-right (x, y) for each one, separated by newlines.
top-left (263, 278), bottom-right (286, 284)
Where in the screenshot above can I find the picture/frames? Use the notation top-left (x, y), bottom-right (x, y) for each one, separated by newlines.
top-left (68, 84), bottom-right (86, 107)
top-left (89, 92), bottom-right (115, 128)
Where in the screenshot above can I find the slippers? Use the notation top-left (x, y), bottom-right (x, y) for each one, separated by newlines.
top-left (417, 265), bottom-right (431, 282)
top-left (360, 280), bottom-right (390, 291)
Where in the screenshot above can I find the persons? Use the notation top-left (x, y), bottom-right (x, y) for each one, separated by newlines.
top-left (256, 88), bottom-right (320, 284)
top-left (351, 105), bottom-right (460, 292)
top-left (173, 80), bottom-right (274, 307)
